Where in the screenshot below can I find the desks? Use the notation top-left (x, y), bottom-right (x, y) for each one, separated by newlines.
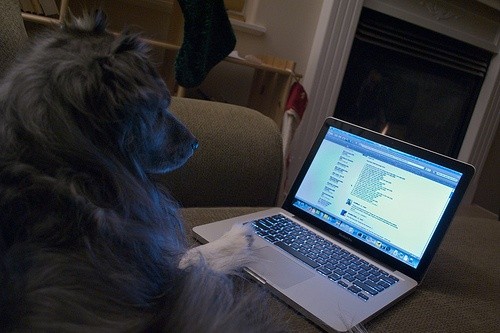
top-left (178, 201), bottom-right (499, 332)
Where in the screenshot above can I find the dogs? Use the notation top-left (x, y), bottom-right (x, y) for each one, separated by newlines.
top-left (0, 6), bottom-right (285, 333)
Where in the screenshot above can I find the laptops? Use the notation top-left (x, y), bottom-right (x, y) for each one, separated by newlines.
top-left (190, 116), bottom-right (477, 333)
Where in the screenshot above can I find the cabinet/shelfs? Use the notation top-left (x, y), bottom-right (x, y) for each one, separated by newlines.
top-left (22, 0), bottom-right (300, 127)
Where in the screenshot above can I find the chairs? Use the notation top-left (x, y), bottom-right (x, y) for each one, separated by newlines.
top-left (1, 0), bottom-right (283, 207)
